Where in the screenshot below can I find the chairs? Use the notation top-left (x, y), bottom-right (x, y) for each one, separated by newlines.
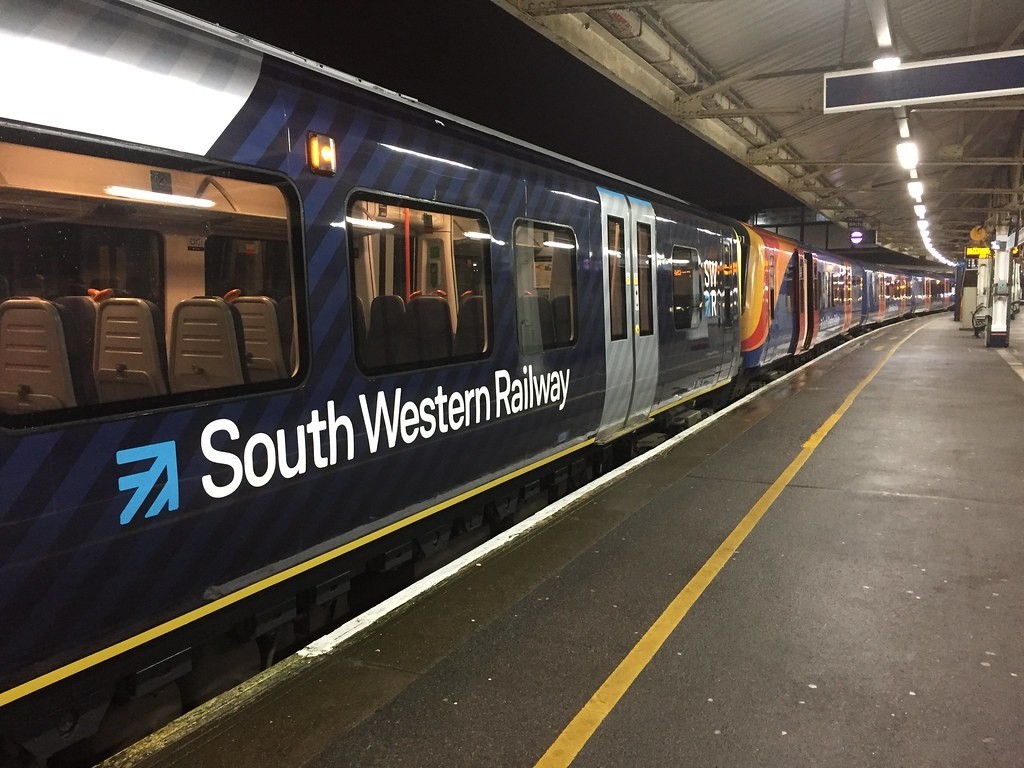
top-left (0, 293), bottom-right (571, 416)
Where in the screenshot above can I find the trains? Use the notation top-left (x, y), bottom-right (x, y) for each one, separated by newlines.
top-left (0, 0), bottom-right (958, 768)
